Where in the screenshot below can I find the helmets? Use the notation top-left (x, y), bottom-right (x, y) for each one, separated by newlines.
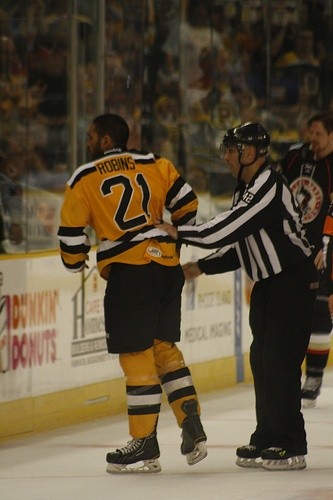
top-left (223, 120), bottom-right (270, 148)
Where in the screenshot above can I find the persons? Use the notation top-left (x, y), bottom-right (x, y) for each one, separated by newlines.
top-left (0, 6), bottom-right (333, 253)
top-left (56, 113), bottom-right (207, 474)
top-left (154, 121), bottom-right (313, 472)
top-left (284, 113), bottom-right (333, 409)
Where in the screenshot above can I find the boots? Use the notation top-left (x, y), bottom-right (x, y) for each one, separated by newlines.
top-left (106, 415), bottom-right (162, 474)
top-left (180, 399), bottom-right (207, 466)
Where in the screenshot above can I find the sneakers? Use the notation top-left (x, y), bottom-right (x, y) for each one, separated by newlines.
top-left (301, 376), bottom-right (322, 409)
top-left (235, 429), bottom-right (307, 471)
